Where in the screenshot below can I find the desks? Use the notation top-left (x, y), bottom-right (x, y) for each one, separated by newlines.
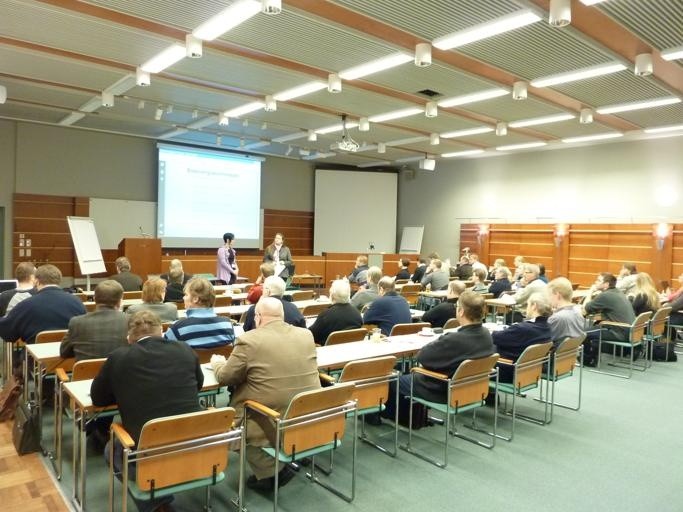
top-left (21, 341), bottom-right (74, 459)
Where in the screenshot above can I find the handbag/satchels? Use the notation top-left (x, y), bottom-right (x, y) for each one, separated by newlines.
top-left (10, 400), bottom-right (43, 457)
top-left (0, 375), bottom-right (23, 422)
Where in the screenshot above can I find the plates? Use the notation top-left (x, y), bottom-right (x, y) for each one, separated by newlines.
top-left (418, 332), bottom-right (434, 336)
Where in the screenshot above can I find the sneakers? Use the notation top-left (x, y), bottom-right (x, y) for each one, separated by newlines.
top-left (358, 413), bottom-right (383, 424)
top-left (137, 495), bottom-right (175, 511)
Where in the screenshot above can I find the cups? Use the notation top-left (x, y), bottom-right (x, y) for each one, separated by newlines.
top-left (422, 327), bottom-right (433, 333)
top-left (372, 328), bottom-right (381, 343)
top-left (425, 286), bottom-right (429, 293)
top-left (335, 274), bottom-right (339, 280)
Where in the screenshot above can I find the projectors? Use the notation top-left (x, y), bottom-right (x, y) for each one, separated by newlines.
top-left (330, 141), bottom-right (356, 154)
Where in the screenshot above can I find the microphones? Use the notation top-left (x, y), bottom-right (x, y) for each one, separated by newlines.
top-left (139, 226), bottom-right (145, 238)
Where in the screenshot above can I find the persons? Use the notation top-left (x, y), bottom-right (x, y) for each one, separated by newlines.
top-left (1, 252), bottom-right (323, 512)
top-left (308, 252), bottom-right (682, 429)
top-left (262, 231), bottom-right (294, 268)
top-left (215, 232), bottom-right (238, 285)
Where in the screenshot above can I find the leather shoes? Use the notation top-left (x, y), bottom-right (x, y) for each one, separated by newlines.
top-left (246, 465), bottom-right (297, 494)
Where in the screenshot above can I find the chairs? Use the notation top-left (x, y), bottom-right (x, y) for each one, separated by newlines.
top-left (461, 340), bottom-right (551, 443)
top-left (398, 353), bottom-right (500, 470)
top-left (48, 356), bottom-right (110, 483)
top-left (238, 381), bottom-right (359, 512)
top-left (106, 402), bottom-right (246, 512)
top-left (504, 331), bottom-right (586, 425)
top-left (302, 355), bottom-right (400, 475)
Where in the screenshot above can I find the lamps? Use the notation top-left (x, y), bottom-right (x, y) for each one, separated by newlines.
top-left (653, 222), bottom-right (667, 252)
top-left (472, 221), bottom-right (492, 247)
top-left (551, 222), bottom-right (568, 250)
